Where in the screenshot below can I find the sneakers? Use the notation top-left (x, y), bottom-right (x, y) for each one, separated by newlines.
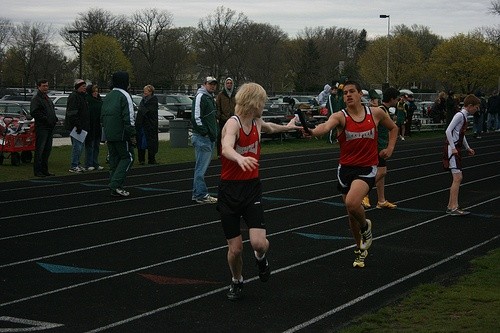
top-left (254, 251), bottom-right (271, 279)
top-left (228, 276), bottom-right (244, 299)
top-left (376, 200), bottom-right (397, 208)
top-left (359, 219), bottom-right (372, 250)
top-left (196, 195), bottom-right (217, 203)
top-left (108, 186), bottom-right (130, 196)
top-left (78, 165), bottom-right (86, 172)
top-left (87, 166), bottom-right (94, 171)
top-left (192, 195), bottom-right (196, 201)
top-left (96, 165), bottom-right (104, 169)
top-left (353, 250), bottom-right (368, 268)
top-left (446, 207), bottom-right (470, 215)
top-left (361, 195), bottom-right (370, 208)
top-left (69, 166), bottom-right (83, 172)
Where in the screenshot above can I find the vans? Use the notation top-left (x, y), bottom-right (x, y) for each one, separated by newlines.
top-left (359, 89), bottom-right (396, 116)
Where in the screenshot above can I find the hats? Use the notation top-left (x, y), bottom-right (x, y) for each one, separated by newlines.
top-left (330, 85), bottom-right (337, 90)
top-left (205, 76), bottom-right (217, 83)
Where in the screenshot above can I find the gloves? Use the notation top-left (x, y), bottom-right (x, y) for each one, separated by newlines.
top-left (76, 124), bottom-right (82, 134)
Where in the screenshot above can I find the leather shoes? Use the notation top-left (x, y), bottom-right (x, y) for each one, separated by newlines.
top-left (46, 172), bottom-right (56, 176)
top-left (36, 173), bottom-right (46, 177)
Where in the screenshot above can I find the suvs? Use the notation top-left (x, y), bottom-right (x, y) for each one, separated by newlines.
top-left (264, 96), bottom-right (321, 112)
top-left (0, 88), bottom-right (176, 137)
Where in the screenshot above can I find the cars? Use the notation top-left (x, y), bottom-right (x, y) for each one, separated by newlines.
top-left (138, 94), bottom-right (200, 133)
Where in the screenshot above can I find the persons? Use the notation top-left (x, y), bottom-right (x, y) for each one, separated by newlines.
top-left (444, 95), bottom-right (481, 217)
top-left (101, 72), bottom-right (135, 197)
top-left (362, 87), bottom-right (400, 210)
top-left (316, 82), bottom-right (415, 142)
top-left (30, 78), bottom-right (59, 177)
top-left (84, 84), bottom-right (104, 171)
top-left (217, 77), bottom-right (237, 164)
top-left (421, 89), bottom-right (500, 140)
top-left (215, 81), bottom-right (304, 301)
top-left (135, 85), bottom-right (161, 165)
top-left (192, 77), bottom-right (219, 203)
top-left (64, 79), bottom-right (87, 173)
top-left (302, 80), bottom-right (399, 267)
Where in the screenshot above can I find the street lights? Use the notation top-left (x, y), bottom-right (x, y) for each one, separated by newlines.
top-left (379, 15), bottom-right (391, 85)
top-left (67, 30), bottom-right (95, 80)
